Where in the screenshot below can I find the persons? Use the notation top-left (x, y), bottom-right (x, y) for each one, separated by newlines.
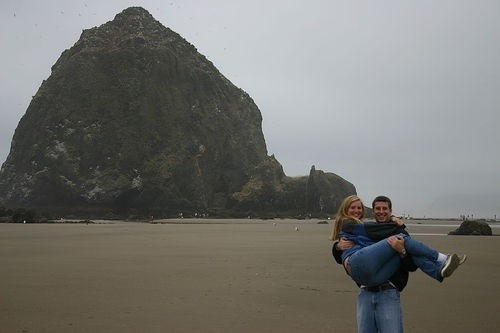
top-left (331, 194), bottom-right (421, 333)
top-left (329, 194), bottom-right (467, 290)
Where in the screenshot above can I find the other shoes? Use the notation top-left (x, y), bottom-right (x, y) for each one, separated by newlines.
top-left (446, 252), bottom-right (466, 269)
top-left (440, 254), bottom-right (459, 278)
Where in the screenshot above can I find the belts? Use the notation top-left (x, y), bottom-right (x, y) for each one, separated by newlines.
top-left (361, 284), bottom-right (398, 293)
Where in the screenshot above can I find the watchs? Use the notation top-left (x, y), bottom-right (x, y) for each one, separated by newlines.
top-left (399, 249), bottom-right (407, 255)
